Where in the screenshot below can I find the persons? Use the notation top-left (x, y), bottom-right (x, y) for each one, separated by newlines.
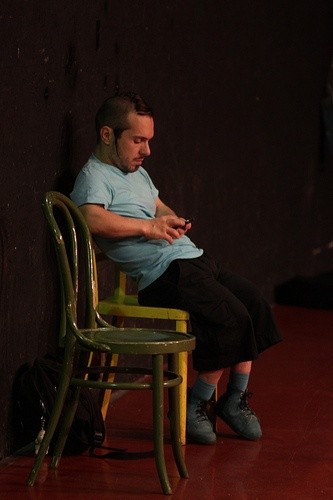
top-left (69, 90), bottom-right (284, 445)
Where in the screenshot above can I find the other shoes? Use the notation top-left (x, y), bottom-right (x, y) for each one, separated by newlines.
top-left (214, 387), bottom-right (262, 440)
top-left (187, 387), bottom-right (216, 443)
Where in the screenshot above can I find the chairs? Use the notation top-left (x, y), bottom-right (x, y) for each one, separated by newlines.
top-left (27, 192), bottom-right (187, 495)
top-left (95, 218), bottom-right (191, 445)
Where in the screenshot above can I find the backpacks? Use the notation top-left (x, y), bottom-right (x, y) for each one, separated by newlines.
top-left (8, 354), bottom-right (105, 457)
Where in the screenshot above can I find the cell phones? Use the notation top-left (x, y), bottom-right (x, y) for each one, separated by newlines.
top-left (172, 218), bottom-right (193, 229)
